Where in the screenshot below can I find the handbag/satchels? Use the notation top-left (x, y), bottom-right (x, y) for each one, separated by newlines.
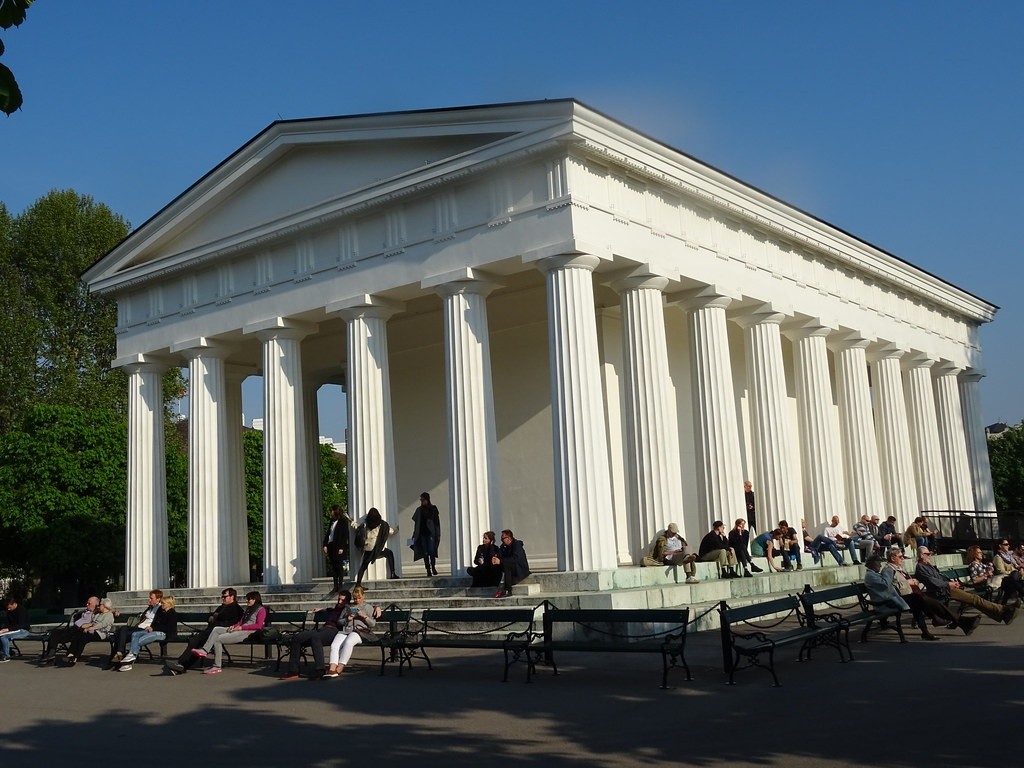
top-left (243, 626), bottom-right (280, 643)
top-left (355, 623), bottom-right (379, 644)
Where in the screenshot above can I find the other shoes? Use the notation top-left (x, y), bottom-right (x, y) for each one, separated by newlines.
top-left (38, 651), bottom-right (55, 666)
top-left (161, 659), bottom-right (184, 676)
top-left (682, 555), bottom-right (696, 564)
top-left (202, 666), bottom-right (222, 674)
top-left (118, 653), bottom-right (136, 672)
top-left (920, 598), bottom-right (1021, 641)
top-left (835, 543), bottom-right (846, 549)
top-left (782, 565), bottom-right (793, 572)
top-left (111, 651), bottom-right (125, 662)
top-left (191, 648), bottom-right (207, 658)
top-left (796, 563), bottom-right (803, 571)
top-left (853, 560), bottom-right (862, 564)
top-left (0, 656), bottom-right (10, 663)
top-left (750, 562), bottom-right (763, 572)
top-left (495, 588), bottom-right (512, 598)
top-left (721, 567), bottom-right (742, 579)
top-left (839, 561), bottom-right (851, 566)
top-left (323, 671), bottom-right (341, 677)
top-left (685, 576), bottom-right (699, 583)
top-left (744, 568), bottom-right (753, 577)
top-left (280, 672), bottom-right (299, 680)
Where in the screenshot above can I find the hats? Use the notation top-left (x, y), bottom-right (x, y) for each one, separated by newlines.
top-left (668, 523), bottom-right (679, 533)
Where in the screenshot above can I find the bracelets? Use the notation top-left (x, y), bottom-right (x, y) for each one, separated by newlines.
top-left (363, 617), bottom-right (368, 620)
top-left (350, 615), bottom-right (353, 618)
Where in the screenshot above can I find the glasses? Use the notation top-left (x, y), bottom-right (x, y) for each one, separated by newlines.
top-left (222, 596), bottom-right (228, 599)
top-left (864, 518), bottom-right (871, 522)
top-left (502, 537), bottom-right (508, 540)
top-left (1001, 543), bottom-right (1010, 546)
top-left (923, 553), bottom-right (931, 556)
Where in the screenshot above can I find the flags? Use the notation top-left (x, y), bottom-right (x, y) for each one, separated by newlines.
top-left (180, 387), bottom-right (187, 399)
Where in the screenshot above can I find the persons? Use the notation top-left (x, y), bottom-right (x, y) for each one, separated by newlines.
top-left (344, 508), bottom-right (401, 592)
top-left (39, 590), bottom-right (178, 671)
top-left (411, 492), bottom-right (440, 577)
top-left (323, 504), bottom-right (349, 594)
top-left (744, 481), bottom-right (757, 536)
top-left (467, 532), bottom-right (503, 587)
top-left (280, 587), bottom-right (381, 680)
top-left (164, 588), bottom-right (244, 675)
top-left (657, 515), bottom-right (1024, 641)
top-left (0, 597), bottom-right (32, 664)
top-left (492, 530), bottom-right (530, 596)
top-left (191, 591), bottom-right (266, 674)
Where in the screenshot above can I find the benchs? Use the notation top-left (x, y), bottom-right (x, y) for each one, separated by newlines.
top-left (16, 600), bottom-right (693, 688)
top-left (718, 567), bottom-right (989, 688)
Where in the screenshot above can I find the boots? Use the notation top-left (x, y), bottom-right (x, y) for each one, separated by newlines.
top-left (389, 557), bottom-right (400, 579)
top-left (357, 568), bottom-right (369, 590)
top-left (431, 558), bottom-right (438, 575)
top-left (425, 564), bottom-right (432, 577)
